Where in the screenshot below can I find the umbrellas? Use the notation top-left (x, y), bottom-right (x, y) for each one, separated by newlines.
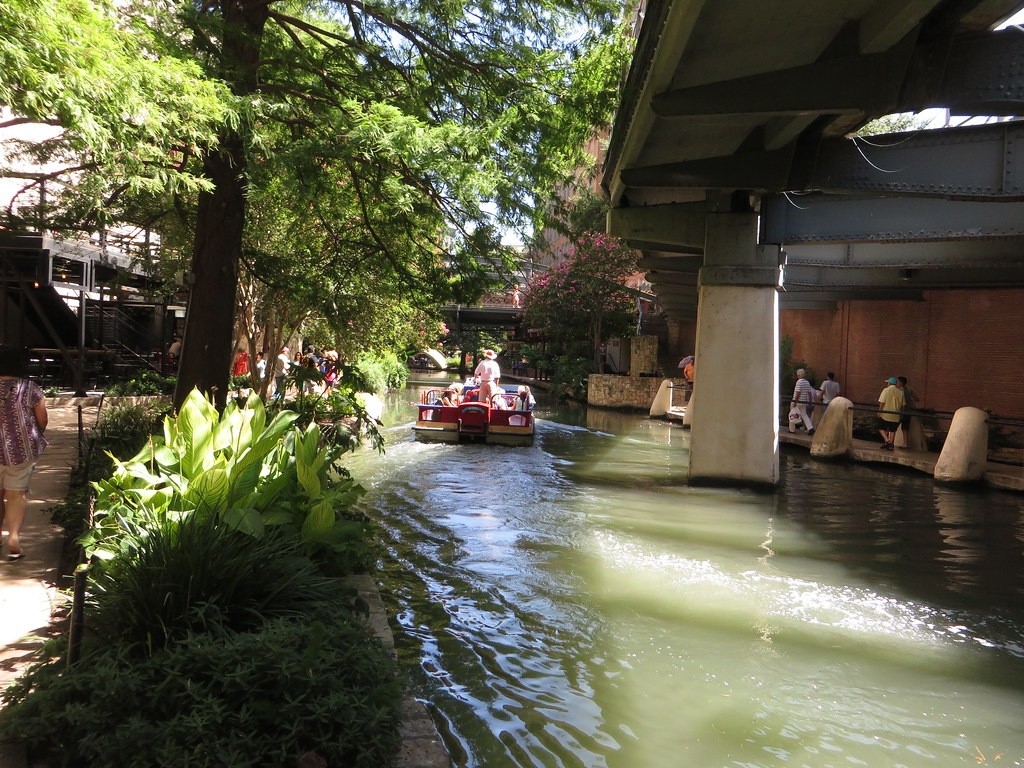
top-left (678, 355), bottom-right (694, 368)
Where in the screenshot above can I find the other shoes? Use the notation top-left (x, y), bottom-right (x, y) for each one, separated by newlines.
top-left (788, 431), bottom-right (795, 434)
top-left (808, 428), bottom-right (815, 436)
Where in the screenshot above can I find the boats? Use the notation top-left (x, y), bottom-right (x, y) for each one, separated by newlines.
top-left (409, 380), bottom-right (536, 446)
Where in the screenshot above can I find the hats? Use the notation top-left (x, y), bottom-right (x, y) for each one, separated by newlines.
top-left (307, 345), bottom-right (314, 350)
top-left (885, 377), bottom-right (896, 384)
top-left (257, 352), bottom-right (263, 356)
top-left (485, 350), bottom-right (497, 359)
top-left (282, 347), bottom-right (289, 351)
top-left (441, 388), bottom-right (454, 392)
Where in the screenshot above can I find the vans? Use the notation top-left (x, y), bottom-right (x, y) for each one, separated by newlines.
top-left (27, 349), bottom-right (99, 382)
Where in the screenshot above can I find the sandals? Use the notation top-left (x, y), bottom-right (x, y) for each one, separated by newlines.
top-left (886, 446), bottom-right (893, 451)
top-left (881, 440), bottom-right (891, 449)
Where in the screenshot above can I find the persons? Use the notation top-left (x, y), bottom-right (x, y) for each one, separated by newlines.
top-left (441, 382), bottom-right (461, 406)
top-left (512, 360), bottom-right (523, 376)
top-left (495, 386), bottom-right (535, 409)
top-left (0, 346), bottom-right (48, 558)
top-left (272, 347), bottom-right (290, 400)
top-left (291, 346), bottom-right (338, 398)
top-left (786, 369), bottom-right (814, 435)
top-left (877, 377), bottom-right (905, 451)
top-left (167, 336), bottom-right (182, 358)
top-left (234, 349), bottom-right (248, 376)
top-left (513, 285), bottom-right (520, 308)
top-left (256, 352), bottom-right (265, 381)
top-left (897, 376), bottom-right (920, 449)
top-left (474, 349), bottom-right (500, 404)
top-left (816, 372), bottom-right (840, 410)
top-left (683, 358), bottom-right (694, 401)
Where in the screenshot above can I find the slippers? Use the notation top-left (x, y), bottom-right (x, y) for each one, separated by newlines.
top-left (7, 547), bottom-right (26, 559)
